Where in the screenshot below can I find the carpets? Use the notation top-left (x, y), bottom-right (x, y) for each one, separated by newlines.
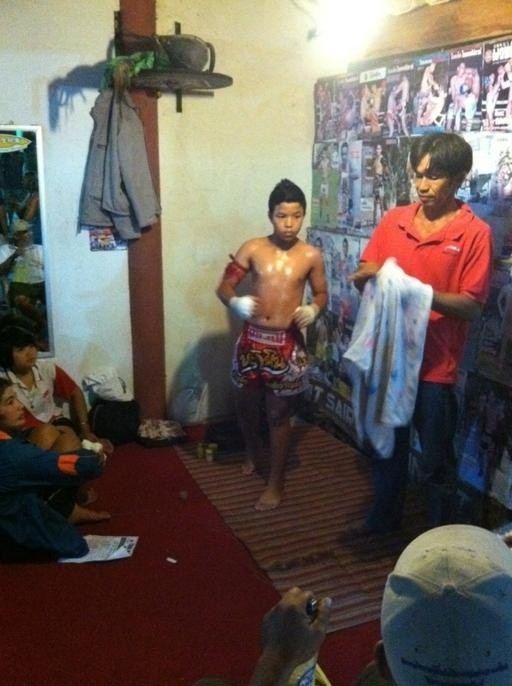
top-left (173, 414), bottom-right (436, 635)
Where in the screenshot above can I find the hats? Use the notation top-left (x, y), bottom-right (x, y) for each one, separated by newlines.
top-left (12, 219), bottom-right (36, 235)
top-left (381, 524), bottom-right (511, 686)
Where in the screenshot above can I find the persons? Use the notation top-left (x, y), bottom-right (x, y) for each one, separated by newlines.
top-left (0, 377), bottom-right (112, 558)
top-left (0, 172), bottom-right (46, 313)
top-left (248, 522), bottom-right (511, 686)
top-left (0, 327), bottom-right (114, 455)
top-left (347, 132), bottom-right (494, 535)
top-left (216, 178), bottom-right (328, 511)
top-left (306, 43), bottom-right (511, 492)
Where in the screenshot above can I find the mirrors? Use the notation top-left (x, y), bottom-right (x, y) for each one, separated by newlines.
top-left (0, 125), bottom-right (54, 361)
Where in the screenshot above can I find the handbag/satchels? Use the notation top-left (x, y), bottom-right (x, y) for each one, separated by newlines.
top-left (87, 400), bottom-right (140, 444)
